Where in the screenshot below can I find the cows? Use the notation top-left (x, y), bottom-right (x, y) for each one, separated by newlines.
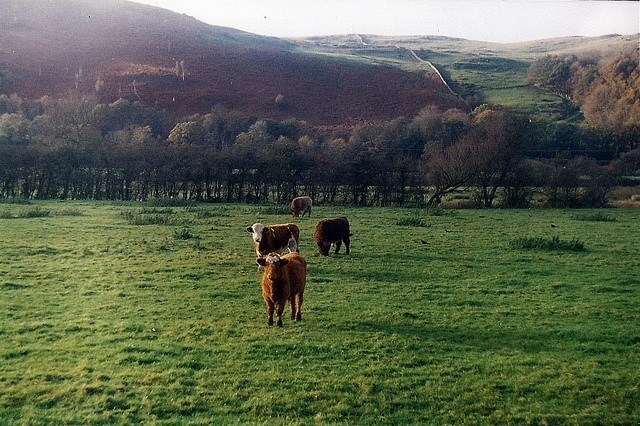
top-left (244, 223), bottom-right (299, 272)
top-left (314, 217), bottom-right (350, 255)
top-left (256, 252), bottom-right (307, 327)
top-left (289, 197), bottom-right (312, 218)
